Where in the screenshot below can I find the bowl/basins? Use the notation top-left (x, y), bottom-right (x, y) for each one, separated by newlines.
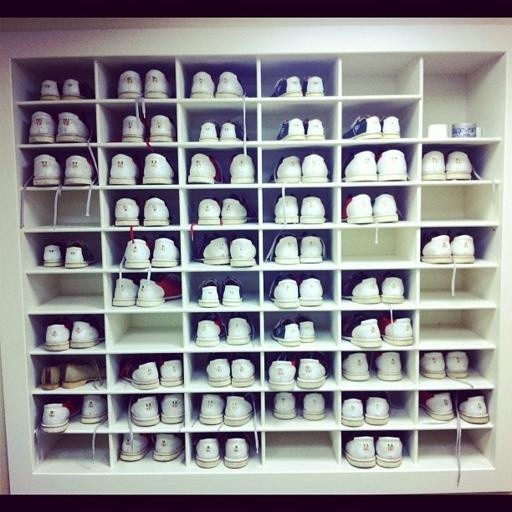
top-left (451, 122), bottom-right (476, 138)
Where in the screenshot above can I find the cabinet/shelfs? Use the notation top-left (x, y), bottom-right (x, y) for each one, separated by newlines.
top-left (0, 16), bottom-right (512, 499)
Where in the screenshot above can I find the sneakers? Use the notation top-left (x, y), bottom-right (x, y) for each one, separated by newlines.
top-left (221, 194), bottom-right (255, 224)
top-left (62, 359), bottom-right (105, 389)
top-left (198, 120), bottom-right (221, 142)
top-left (61, 78), bottom-right (94, 100)
top-left (143, 153), bottom-right (175, 184)
top-left (143, 198), bottom-right (170, 227)
top-left (194, 313), bottom-right (227, 346)
top-left (302, 154), bottom-right (332, 183)
top-left (452, 229), bottom-right (475, 263)
top-left (265, 232), bottom-right (300, 265)
top-left (109, 153), bottom-right (138, 185)
top-left (154, 434), bottom-right (183, 464)
top-left (120, 434), bottom-right (150, 462)
top-left (65, 240), bottom-right (96, 268)
top-left (277, 118), bottom-right (306, 141)
top-left (220, 120), bottom-right (249, 142)
top-left (29, 111), bottom-right (57, 144)
top-left (43, 240), bottom-right (65, 266)
top-left (292, 314), bottom-right (318, 343)
top-left (150, 238), bottom-right (180, 267)
top-left (70, 321), bottom-right (105, 348)
top-left (342, 114), bottom-right (382, 139)
top-left (124, 395), bottom-right (161, 440)
top-left (157, 357), bottom-right (184, 387)
top-left (365, 392), bottom-right (391, 425)
top-left (420, 352), bottom-right (446, 378)
top-left (149, 115), bottom-right (177, 142)
top-left (271, 316), bottom-right (301, 347)
top-left (117, 70), bottom-right (144, 99)
top-left (231, 359), bottom-right (256, 388)
top-left (121, 116), bottom-right (146, 143)
top-left (378, 312), bottom-right (414, 345)
top-left (32, 154), bottom-right (64, 186)
top-left (161, 394), bottom-right (184, 432)
top-left (376, 437), bottom-right (403, 468)
top-left (422, 151), bottom-right (447, 180)
top-left (303, 118), bottom-right (325, 140)
top-left (342, 151), bottom-right (378, 182)
top-left (119, 239), bottom-right (151, 270)
top-left (297, 352), bottom-right (332, 389)
top-left (268, 156), bottom-right (302, 183)
top-left (64, 155), bottom-right (93, 186)
top-left (381, 277), bottom-right (404, 305)
top-left (300, 196), bottom-right (328, 224)
top-left (39, 78), bottom-right (62, 100)
top-left (301, 75), bottom-right (325, 97)
top-left (297, 393), bottom-right (326, 421)
top-left (372, 192), bottom-right (398, 224)
top-left (40, 395), bottom-right (83, 433)
top-left (142, 69), bottom-right (169, 99)
top-left (269, 76), bottom-right (303, 97)
top-left (275, 193), bottom-right (300, 225)
top-left (226, 313), bottom-right (256, 346)
top-left (115, 198), bottom-right (140, 226)
top-left (457, 390), bottom-right (489, 425)
top-left (273, 393), bottom-right (297, 419)
top-left (446, 151), bottom-right (473, 181)
top-left (342, 192), bottom-right (374, 223)
top-left (81, 395), bottom-right (108, 424)
top-left (56, 112), bottom-right (91, 141)
top-left (192, 235), bottom-right (230, 265)
top-left (119, 359), bottom-right (160, 389)
top-left (193, 437), bottom-right (222, 468)
top-left (136, 273), bottom-right (182, 307)
top-left (419, 391), bottom-right (455, 421)
top-left (369, 352), bottom-right (403, 381)
top-left (190, 71), bottom-right (216, 98)
top-left (342, 272), bottom-right (381, 304)
top-left (421, 231), bottom-right (453, 263)
top-left (342, 315), bottom-right (382, 348)
top-left (267, 274), bottom-right (300, 309)
top-left (40, 362), bottom-right (64, 390)
top-left (222, 276), bottom-right (247, 306)
top-left (215, 71), bottom-right (247, 99)
top-left (380, 116), bottom-right (401, 139)
top-left (342, 437), bottom-right (376, 467)
top-left (198, 394), bottom-right (225, 425)
top-left (444, 352), bottom-right (470, 377)
top-left (266, 352), bottom-right (299, 391)
top-left (377, 150), bottom-right (412, 181)
top-left (223, 437), bottom-right (251, 468)
top-left (343, 353), bottom-right (370, 381)
top-left (45, 324), bottom-right (72, 350)
top-left (188, 153), bottom-right (225, 184)
top-left (230, 238), bottom-right (257, 267)
top-left (223, 392), bottom-right (258, 427)
top-left (300, 231), bottom-right (328, 263)
top-left (112, 278), bottom-right (139, 306)
top-left (342, 399), bottom-right (364, 427)
top-left (299, 273), bottom-right (323, 306)
top-left (207, 359), bottom-right (232, 387)
top-left (229, 154), bottom-right (256, 184)
top-left (196, 197), bottom-right (221, 225)
top-left (198, 278), bottom-right (221, 308)
